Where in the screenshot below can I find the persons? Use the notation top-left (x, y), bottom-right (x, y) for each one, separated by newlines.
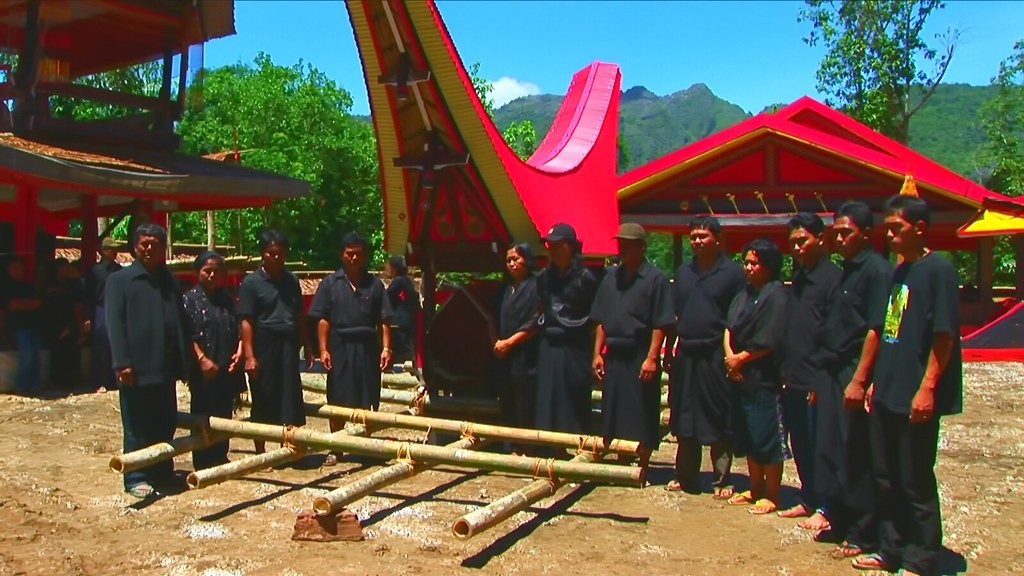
top-left (852, 195), bottom-right (964, 576)
top-left (537, 225), bottom-right (598, 436)
top-left (780, 210), bottom-right (844, 530)
top-left (308, 236), bottom-right (394, 466)
top-left (42, 258), bottom-right (82, 386)
top-left (74, 237), bottom-right (124, 394)
top-left (0, 255), bottom-right (50, 391)
top-left (238, 230), bottom-right (315, 463)
top-left (104, 223), bottom-right (182, 499)
top-left (722, 238), bottom-right (797, 516)
top-left (488, 242), bottom-right (545, 424)
top-left (663, 215), bottom-right (749, 501)
top-left (178, 251), bottom-right (243, 471)
top-left (589, 222), bottom-right (678, 470)
top-left (814, 200), bottom-right (890, 555)
top-left (382, 256), bottom-right (417, 361)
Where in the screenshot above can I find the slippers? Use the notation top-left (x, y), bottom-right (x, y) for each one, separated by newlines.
top-left (728, 493), bottom-right (760, 505)
top-left (748, 498), bottom-right (781, 514)
top-left (663, 478), bottom-right (694, 491)
top-left (712, 484), bottom-right (735, 500)
top-left (835, 540), bottom-right (873, 556)
top-left (125, 484), bottom-right (158, 498)
top-left (852, 552), bottom-right (892, 569)
top-left (322, 452), bottom-right (342, 466)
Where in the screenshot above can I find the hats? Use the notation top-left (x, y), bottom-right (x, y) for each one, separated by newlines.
top-left (614, 222), bottom-right (648, 241)
top-left (538, 223), bottom-right (576, 242)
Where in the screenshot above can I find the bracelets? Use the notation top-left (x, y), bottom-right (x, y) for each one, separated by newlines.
top-left (197, 352), bottom-right (204, 361)
top-left (384, 348), bottom-right (393, 354)
